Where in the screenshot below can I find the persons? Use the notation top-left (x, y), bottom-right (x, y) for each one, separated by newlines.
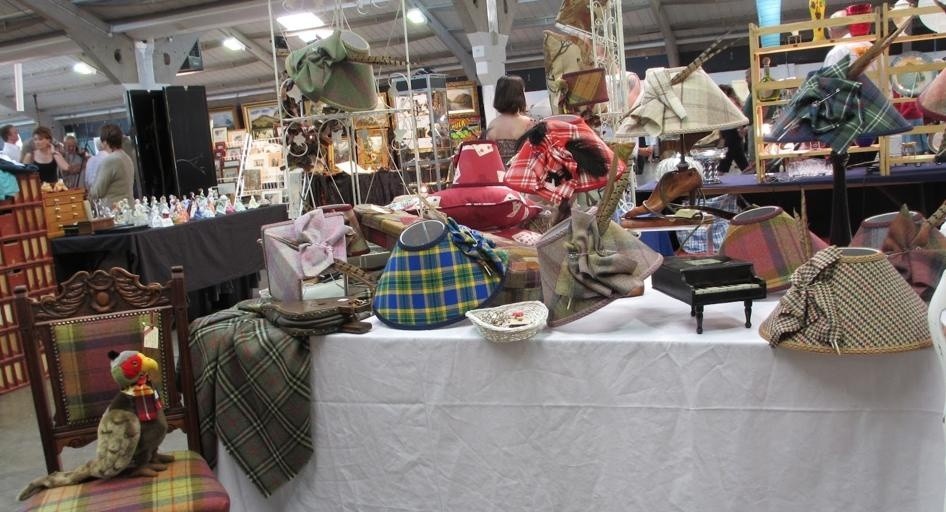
top-left (111, 187), bottom-right (270, 228)
top-left (822, 9), bottom-right (894, 167)
top-left (743, 69), bottom-right (756, 167)
top-left (716, 84), bottom-right (750, 176)
top-left (486, 75), bottom-right (536, 167)
top-left (0, 125), bottom-right (139, 214)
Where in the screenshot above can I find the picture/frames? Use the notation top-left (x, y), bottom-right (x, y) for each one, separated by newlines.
top-left (389, 80), bottom-right (482, 153)
top-left (208, 100), bottom-right (287, 139)
top-left (304, 92), bottom-right (390, 173)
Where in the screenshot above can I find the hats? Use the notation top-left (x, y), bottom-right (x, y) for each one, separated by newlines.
top-left (535, 204), bottom-right (664, 329)
top-left (284, 29), bottom-right (380, 112)
top-left (502, 113), bottom-right (627, 205)
top-left (718, 205), bottom-right (946, 357)
top-left (762, 44), bottom-right (946, 156)
top-left (614, 63), bottom-right (750, 140)
top-left (371, 216), bottom-right (510, 331)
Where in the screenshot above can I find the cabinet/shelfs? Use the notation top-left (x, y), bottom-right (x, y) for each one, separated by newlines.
top-left (0, 167), bottom-right (87, 396)
top-left (748, 3), bottom-right (946, 183)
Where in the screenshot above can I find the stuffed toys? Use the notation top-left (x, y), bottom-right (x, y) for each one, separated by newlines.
top-left (18, 349), bottom-right (174, 502)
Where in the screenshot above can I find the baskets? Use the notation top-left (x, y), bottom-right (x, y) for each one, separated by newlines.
top-left (465, 300), bottom-right (549, 344)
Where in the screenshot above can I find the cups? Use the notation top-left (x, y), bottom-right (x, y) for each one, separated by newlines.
top-left (847, 3), bottom-right (873, 36)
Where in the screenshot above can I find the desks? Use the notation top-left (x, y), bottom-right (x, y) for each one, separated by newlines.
top-left (49, 203), bottom-right (287, 329)
top-left (637, 161), bottom-right (946, 247)
top-left (185, 275), bottom-right (945, 511)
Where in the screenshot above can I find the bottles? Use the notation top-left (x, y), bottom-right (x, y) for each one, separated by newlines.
top-left (756, 57), bottom-right (779, 100)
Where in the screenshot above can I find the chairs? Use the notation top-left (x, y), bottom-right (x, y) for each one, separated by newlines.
top-left (13, 267), bottom-right (231, 512)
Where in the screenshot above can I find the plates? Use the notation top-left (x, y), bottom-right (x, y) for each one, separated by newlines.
top-left (889, 53), bottom-right (937, 97)
top-left (927, 133), bottom-right (943, 154)
top-left (919, 0), bottom-right (946, 34)
